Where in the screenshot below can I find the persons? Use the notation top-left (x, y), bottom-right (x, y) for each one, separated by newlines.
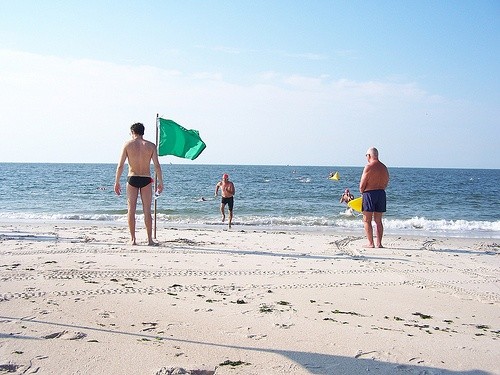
top-left (359, 147), bottom-right (389, 248)
top-left (114, 123), bottom-right (164, 246)
top-left (199, 197), bottom-right (205, 201)
top-left (215, 174), bottom-right (235, 227)
top-left (328, 171), bottom-right (334, 178)
top-left (340, 189), bottom-right (354, 203)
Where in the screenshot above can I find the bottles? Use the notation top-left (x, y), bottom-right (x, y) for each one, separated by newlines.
top-left (152, 190), bottom-right (161, 199)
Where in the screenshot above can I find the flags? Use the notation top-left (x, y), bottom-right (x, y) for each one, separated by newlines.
top-left (156, 117), bottom-right (207, 160)
